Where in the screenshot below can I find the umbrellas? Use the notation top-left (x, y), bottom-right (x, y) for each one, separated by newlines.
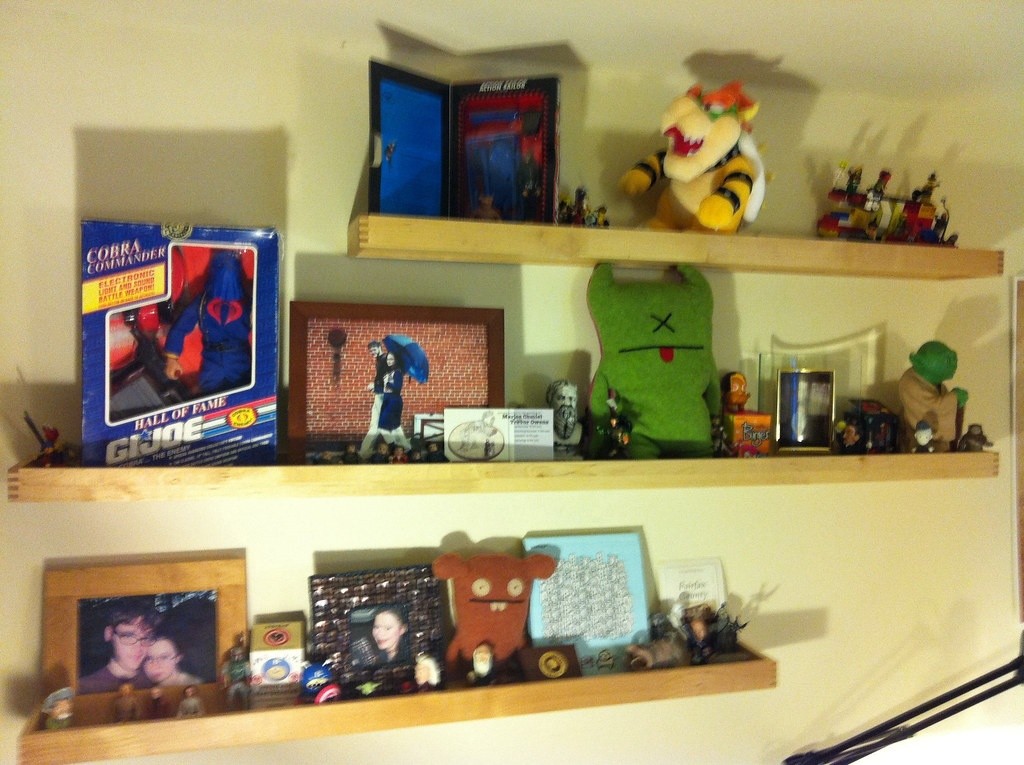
top-left (383, 334), bottom-right (428, 385)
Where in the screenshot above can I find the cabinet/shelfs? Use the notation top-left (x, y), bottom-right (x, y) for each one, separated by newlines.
top-left (6, 213), bottom-right (1006, 765)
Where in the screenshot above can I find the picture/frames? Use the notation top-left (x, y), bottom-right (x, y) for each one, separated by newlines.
top-left (306, 562), bottom-right (451, 702)
top-left (36, 558), bottom-right (247, 728)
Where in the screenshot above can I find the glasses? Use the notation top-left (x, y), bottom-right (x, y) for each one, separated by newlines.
top-left (112, 629), bottom-right (157, 647)
top-left (144, 655), bottom-right (179, 664)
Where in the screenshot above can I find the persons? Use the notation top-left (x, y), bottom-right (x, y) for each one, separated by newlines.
top-left (347, 606), bottom-right (410, 664)
top-left (546, 379), bottom-right (581, 447)
top-left (355, 342), bottom-right (413, 458)
top-left (78, 598), bottom-right (200, 696)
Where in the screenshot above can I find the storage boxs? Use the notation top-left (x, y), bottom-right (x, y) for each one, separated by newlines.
top-left (249, 615), bottom-right (307, 685)
top-left (442, 407), bottom-right (554, 463)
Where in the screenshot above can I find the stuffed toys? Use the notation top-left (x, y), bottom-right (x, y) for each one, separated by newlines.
top-left (431, 549), bottom-right (555, 683)
top-left (618, 77), bottom-right (777, 234)
top-left (585, 263), bottom-right (723, 459)
